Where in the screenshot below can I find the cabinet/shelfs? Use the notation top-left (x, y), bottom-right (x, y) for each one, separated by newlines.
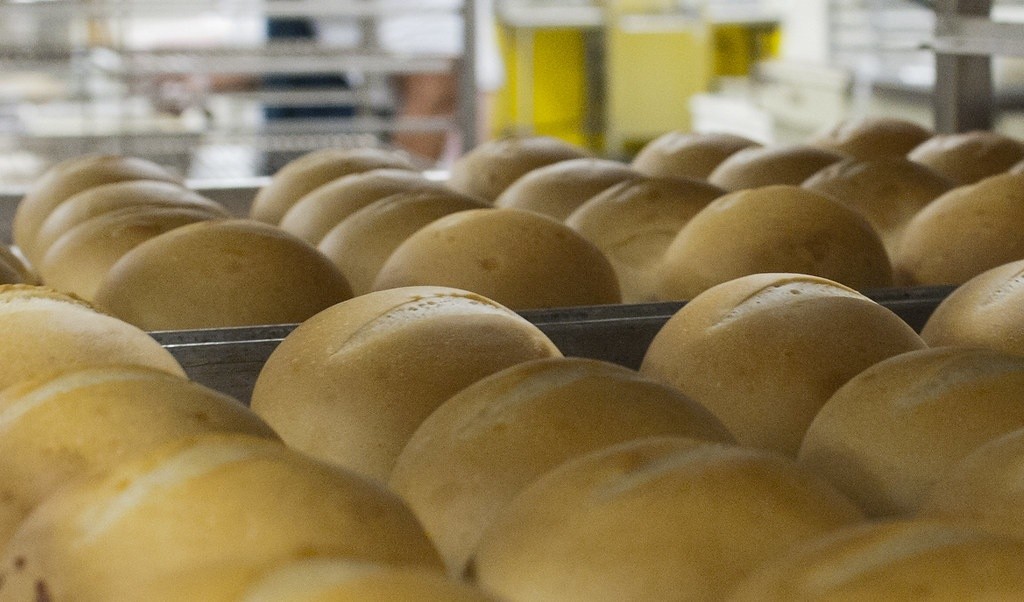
top-left (0, 0), bottom-right (481, 242)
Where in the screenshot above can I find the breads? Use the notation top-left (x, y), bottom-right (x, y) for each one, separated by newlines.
top-left (0, 122), bottom-right (1024, 602)
